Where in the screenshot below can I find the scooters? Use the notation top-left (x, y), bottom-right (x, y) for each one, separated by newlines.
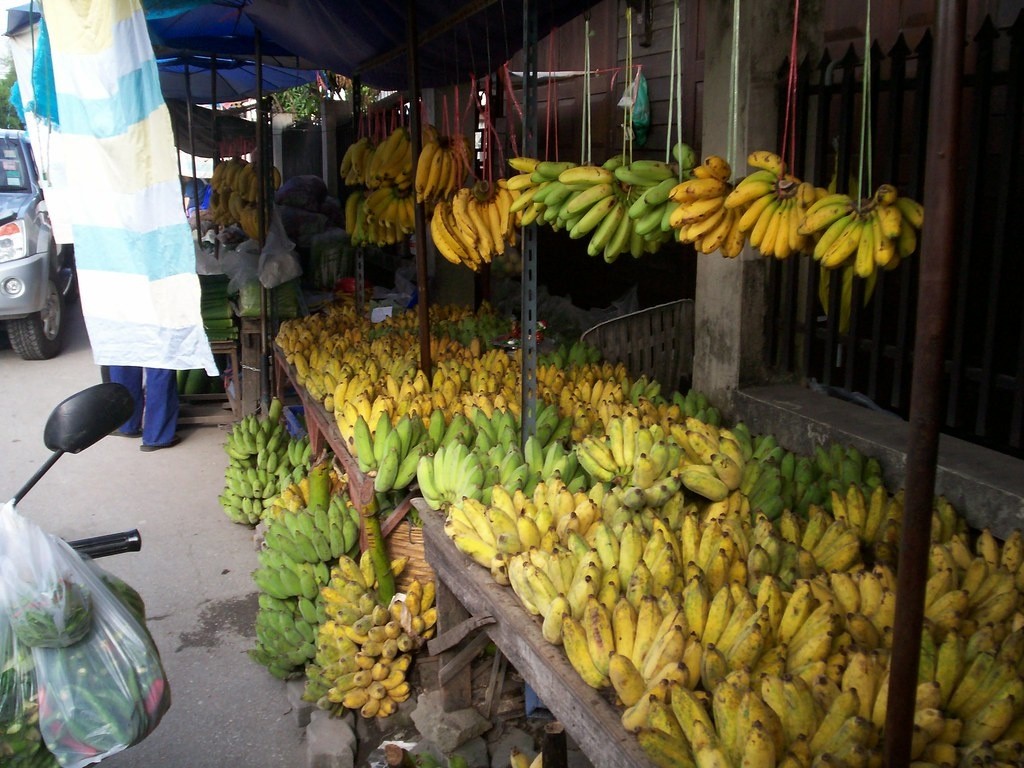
top-left (8, 382), bottom-right (142, 559)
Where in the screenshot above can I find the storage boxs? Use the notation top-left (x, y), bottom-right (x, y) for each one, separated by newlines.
top-left (282, 404), bottom-right (309, 440)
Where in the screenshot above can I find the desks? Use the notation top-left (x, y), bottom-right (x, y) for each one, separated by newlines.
top-left (410, 495), bottom-right (655, 768)
top-left (271, 342), bottom-right (415, 554)
top-left (178, 341), bottom-right (242, 426)
top-left (240, 319), bottom-right (280, 421)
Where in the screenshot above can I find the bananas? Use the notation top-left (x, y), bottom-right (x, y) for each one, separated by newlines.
top-left (209, 158), bottom-right (282, 241)
top-left (276, 296), bottom-right (1024, 768)
top-left (219, 398), bottom-right (438, 719)
top-left (338, 123), bottom-right (525, 272)
top-left (503, 143), bottom-right (924, 277)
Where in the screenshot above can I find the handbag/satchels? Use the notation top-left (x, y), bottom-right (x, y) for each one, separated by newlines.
top-left (0, 501), bottom-right (173, 767)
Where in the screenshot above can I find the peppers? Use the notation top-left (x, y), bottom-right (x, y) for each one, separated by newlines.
top-left (1, 559), bottom-right (171, 767)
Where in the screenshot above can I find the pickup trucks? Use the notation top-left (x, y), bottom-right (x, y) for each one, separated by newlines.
top-left (0, 128), bottom-right (78, 361)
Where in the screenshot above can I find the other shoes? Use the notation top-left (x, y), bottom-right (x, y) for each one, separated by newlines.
top-left (140, 435), bottom-right (181, 452)
top-left (110, 429), bottom-right (143, 438)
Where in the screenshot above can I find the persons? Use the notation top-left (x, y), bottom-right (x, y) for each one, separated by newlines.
top-left (108, 365), bottom-right (182, 452)
top-left (185, 178), bottom-right (212, 218)
